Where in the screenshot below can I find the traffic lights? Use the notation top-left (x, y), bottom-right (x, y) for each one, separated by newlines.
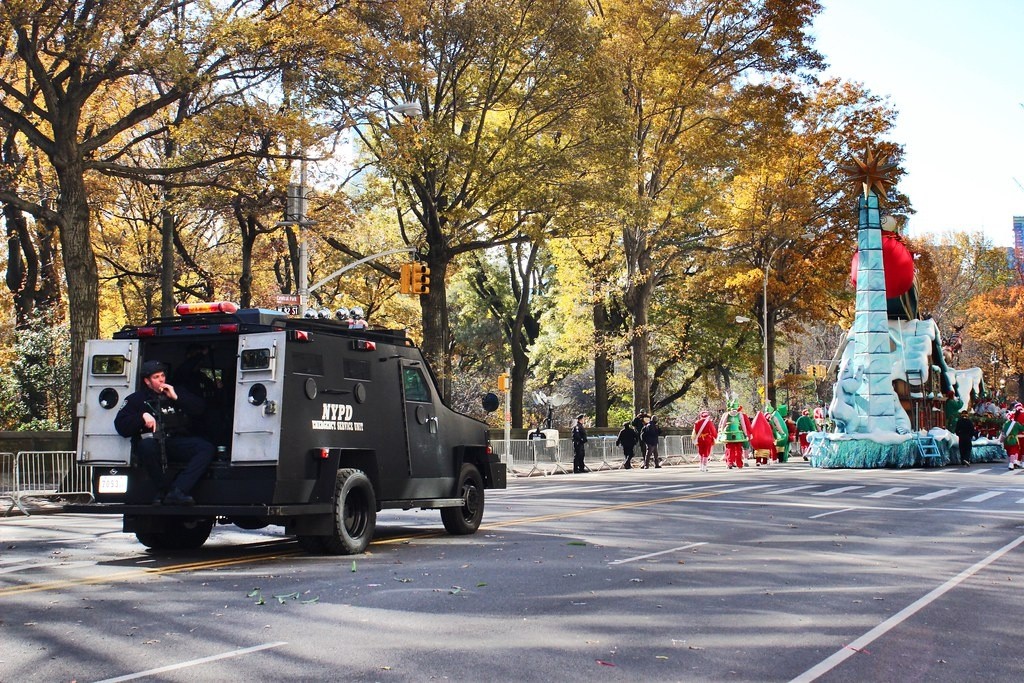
top-left (813, 365), bottom-right (819, 378)
top-left (398, 264), bottom-right (411, 294)
top-left (412, 263), bottom-right (426, 294)
top-left (822, 365), bottom-right (828, 377)
top-left (807, 365), bottom-right (812, 377)
top-left (497, 373), bottom-right (511, 393)
top-left (421, 262), bottom-right (430, 294)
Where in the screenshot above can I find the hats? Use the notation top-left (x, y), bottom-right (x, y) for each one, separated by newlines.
top-left (640, 408), bottom-right (646, 413)
top-left (948, 391), bottom-right (954, 397)
top-left (623, 422), bottom-right (630, 426)
top-left (1006, 414), bottom-right (1014, 420)
top-left (802, 408), bottom-right (810, 416)
top-left (651, 416), bottom-right (658, 421)
top-left (701, 411), bottom-right (708, 418)
top-left (140, 360), bottom-right (167, 378)
top-left (962, 411), bottom-right (968, 414)
top-left (576, 414), bottom-right (583, 419)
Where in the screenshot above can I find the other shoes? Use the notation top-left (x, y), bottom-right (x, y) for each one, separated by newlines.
top-left (964, 460), bottom-right (970, 466)
top-left (1008, 463), bottom-right (1024, 470)
top-left (573, 454), bottom-right (810, 474)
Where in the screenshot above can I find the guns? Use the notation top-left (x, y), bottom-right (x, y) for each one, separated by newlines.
top-left (140, 398), bottom-right (169, 474)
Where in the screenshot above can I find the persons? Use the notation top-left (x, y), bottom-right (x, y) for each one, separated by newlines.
top-left (1000, 402), bottom-right (1024, 470)
top-left (945, 390), bottom-right (964, 434)
top-left (955, 409), bottom-right (974, 468)
top-left (615, 421), bottom-right (639, 470)
top-left (632, 408), bottom-right (662, 470)
top-left (691, 411), bottom-right (718, 472)
top-left (113, 361), bottom-right (215, 506)
top-left (777, 400), bottom-right (834, 463)
top-left (571, 414), bottom-right (588, 474)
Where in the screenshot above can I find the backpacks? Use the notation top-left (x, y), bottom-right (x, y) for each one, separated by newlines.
top-left (639, 424), bottom-right (653, 442)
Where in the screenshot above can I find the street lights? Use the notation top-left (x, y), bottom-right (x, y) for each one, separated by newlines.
top-left (297, 102), bottom-right (424, 317)
top-left (990, 353), bottom-right (999, 391)
top-left (735, 233), bottom-right (817, 412)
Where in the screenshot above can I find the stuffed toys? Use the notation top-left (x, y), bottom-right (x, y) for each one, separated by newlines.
top-left (718, 398), bottom-right (789, 470)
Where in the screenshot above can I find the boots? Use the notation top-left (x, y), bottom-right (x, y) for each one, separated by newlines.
top-left (164, 482), bottom-right (193, 504)
top-left (153, 485), bottom-right (164, 504)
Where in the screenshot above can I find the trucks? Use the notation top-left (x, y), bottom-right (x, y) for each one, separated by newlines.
top-left (74, 301), bottom-right (509, 556)
